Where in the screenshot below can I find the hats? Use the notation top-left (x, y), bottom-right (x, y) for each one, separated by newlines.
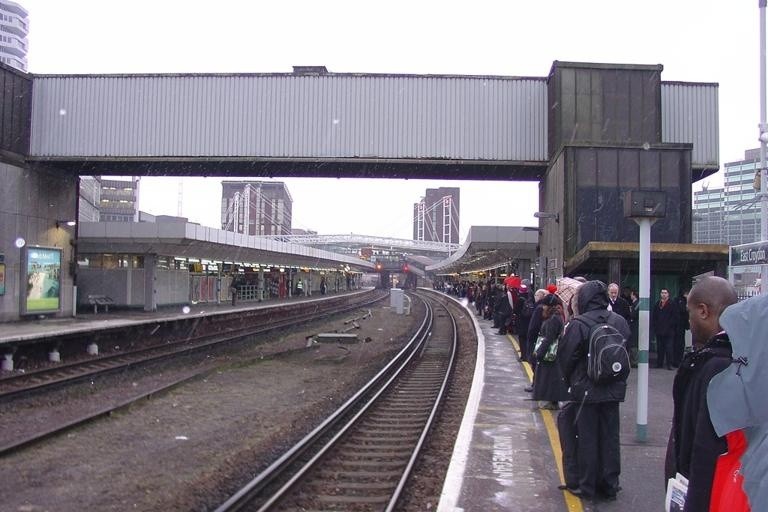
top-left (541, 294), bottom-right (562, 305)
top-left (519, 284), bottom-right (528, 293)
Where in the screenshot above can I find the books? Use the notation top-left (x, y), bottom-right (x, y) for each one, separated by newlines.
top-left (664, 471), bottom-right (691, 512)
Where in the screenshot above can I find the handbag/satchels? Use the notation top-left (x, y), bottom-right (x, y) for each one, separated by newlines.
top-left (533, 335), bottom-right (559, 361)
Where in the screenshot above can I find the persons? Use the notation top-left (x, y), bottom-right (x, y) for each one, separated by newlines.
top-left (434, 272), bottom-right (640, 501)
top-left (229, 277), bottom-right (238, 307)
top-left (319, 276), bottom-right (327, 296)
top-left (663, 277), bottom-right (740, 512)
top-left (295, 278), bottom-right (303, 298)
top-left (325, 277), bottom-right (331, 296)
top-left (705, 289), bottom-right (767, 512)
top-left (650, 287), bottom-right (690, 370)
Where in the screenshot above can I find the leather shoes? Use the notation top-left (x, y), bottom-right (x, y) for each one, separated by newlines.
top-left (567, 487), bottom-right (591, 499)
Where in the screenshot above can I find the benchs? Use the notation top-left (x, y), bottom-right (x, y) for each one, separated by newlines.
top-left (87, 293), bottom-right (116, 316)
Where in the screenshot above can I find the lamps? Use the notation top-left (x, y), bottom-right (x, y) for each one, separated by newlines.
top-left (54, 219), bottom-right (76, 230)
top-left (521, 226), bottom-right (542, 235)
top-left (533, 212), bottom-right (558, 222)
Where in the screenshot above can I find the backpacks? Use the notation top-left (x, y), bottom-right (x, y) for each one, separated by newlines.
top-left (520, 294), bottom-right (534, 316)
top-left (572, 311), bottom-right (631, 383)
